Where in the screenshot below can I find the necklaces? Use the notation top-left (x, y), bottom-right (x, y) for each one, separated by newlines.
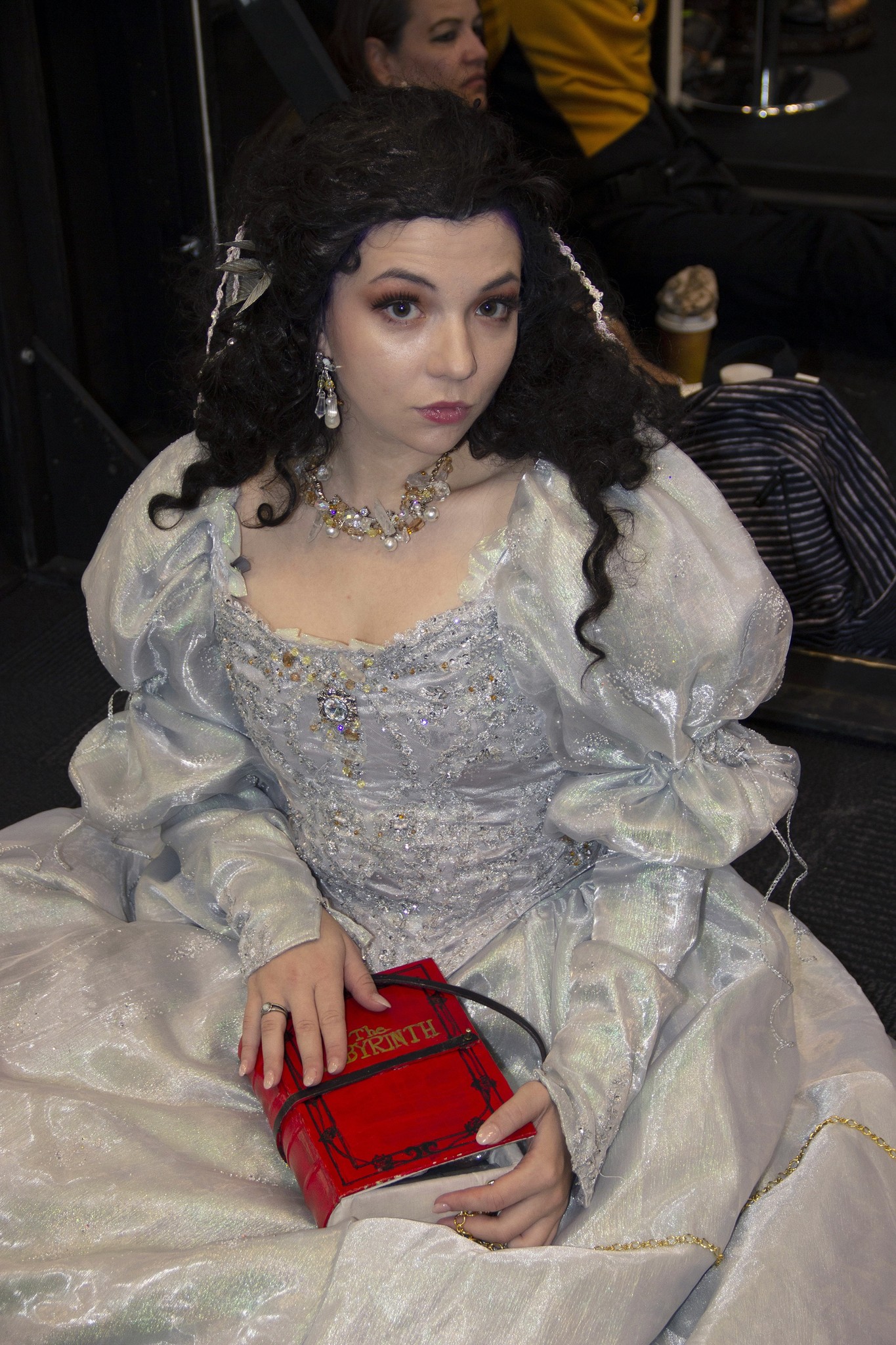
top-left (290, 446), bottom-right (454, 552)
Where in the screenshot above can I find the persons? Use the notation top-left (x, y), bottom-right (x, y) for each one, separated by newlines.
top-left (332, 0), bottom-right (895, 668)
top-left (0, 83), bottom-right (896, 1345)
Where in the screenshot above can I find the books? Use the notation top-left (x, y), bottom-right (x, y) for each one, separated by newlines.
top-left (237, 957), bottom-right (539, 1229)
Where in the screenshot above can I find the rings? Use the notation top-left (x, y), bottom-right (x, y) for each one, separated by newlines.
top-left (260, 1002), bottom-right (288, 1018)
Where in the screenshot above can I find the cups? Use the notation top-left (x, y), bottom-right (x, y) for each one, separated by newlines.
top-left (656, 311), bottom-right (717, 396)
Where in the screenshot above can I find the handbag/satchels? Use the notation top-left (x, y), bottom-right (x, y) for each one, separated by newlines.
top-left (237, 956), bottom-right (529, 1229)
